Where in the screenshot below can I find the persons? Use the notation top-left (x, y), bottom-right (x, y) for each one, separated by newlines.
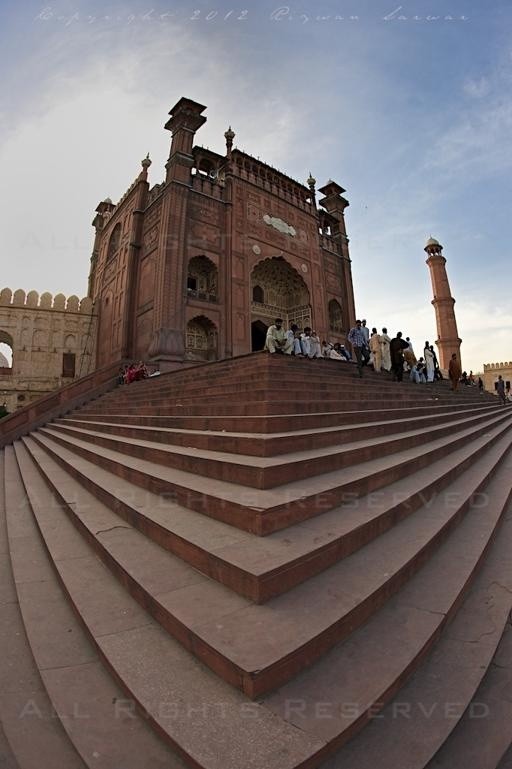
top-left (188, 283), bottom-right (217, 304)
top-left (262, 318), bottom-right (512, 407)
top-left (117, 359), bottom-right (160, 385)
